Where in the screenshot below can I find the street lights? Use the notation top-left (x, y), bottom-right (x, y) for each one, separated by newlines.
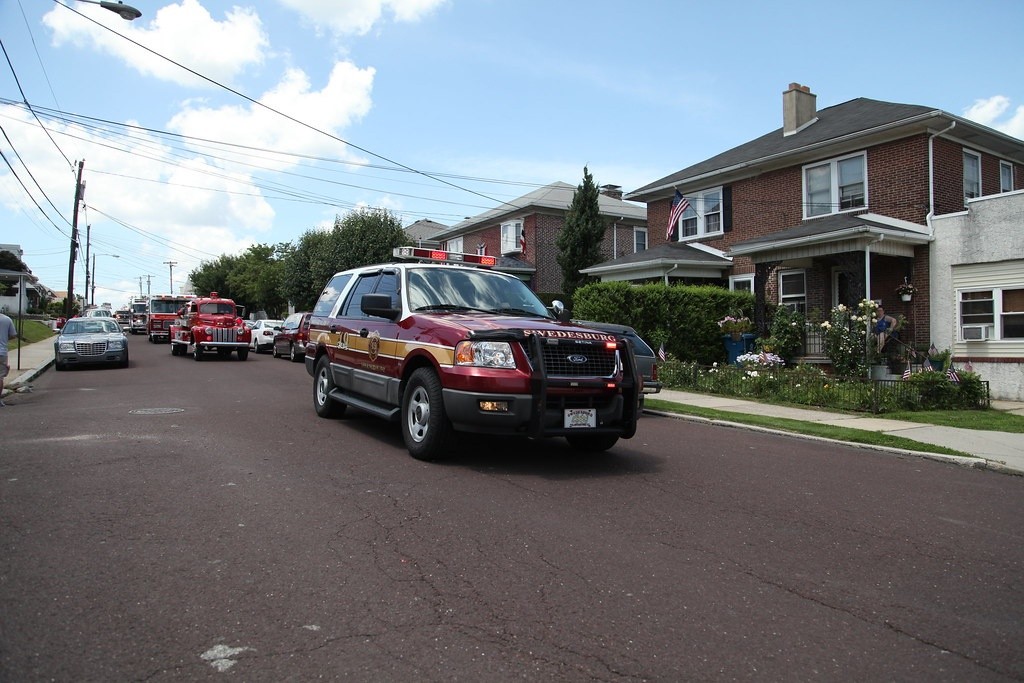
top-left (91, 253), bottom-right (121, 306)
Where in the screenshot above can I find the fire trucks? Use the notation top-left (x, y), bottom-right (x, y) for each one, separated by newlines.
top-left (146, 293), bottom-right (198, 344)
top-left (128, 296), bottom-right (149, 334)
top-left (169, 291), bottom-right (251, 361)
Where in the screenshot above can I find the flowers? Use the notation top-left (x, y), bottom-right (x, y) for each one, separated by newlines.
top-left (895, 283), bottom-right (919, 296)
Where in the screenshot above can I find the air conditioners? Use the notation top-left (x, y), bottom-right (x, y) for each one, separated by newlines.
top-left (961, 324), bottom-right (990, 343)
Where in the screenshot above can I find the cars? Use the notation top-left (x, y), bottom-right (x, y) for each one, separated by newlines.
top-left (80, 309), bottom-right (112, 318)
top-left (53, 316), bottom-right (130, 372)
top-left (246, 319), bottom-right (285, 353)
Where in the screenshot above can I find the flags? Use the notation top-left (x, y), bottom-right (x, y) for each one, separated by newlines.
top-left (665, 187), bottom-right (696, 240)
top-left (520, 223), bottom-right (526, 256)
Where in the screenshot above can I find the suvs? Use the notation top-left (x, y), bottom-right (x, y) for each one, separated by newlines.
top-left (569, 319), bottom-right (662, 395)
top-left (305, 245), bottom-right (645, 462)
top-left (114, 310), bottom-right (129, 324)
top-left (272, 311), bottom-right (312, 361)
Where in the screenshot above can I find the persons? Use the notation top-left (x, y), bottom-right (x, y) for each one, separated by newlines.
top-left (0, 312), bottom-right (18, 407)
top-left (875, 305), bottom-right (896, 356)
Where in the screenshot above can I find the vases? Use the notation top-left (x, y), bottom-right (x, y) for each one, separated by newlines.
top-left (901, 293), bottom-right (912, 301)
